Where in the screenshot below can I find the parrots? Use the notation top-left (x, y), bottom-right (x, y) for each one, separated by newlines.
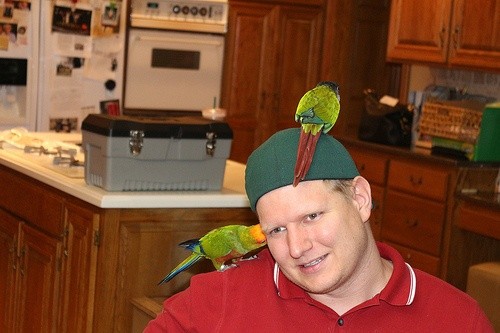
top-left (293, 81), bottom-right (342, 187)
top-left (157, 224), bottom-right (267, 286)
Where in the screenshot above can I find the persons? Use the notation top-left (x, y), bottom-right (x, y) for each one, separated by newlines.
top-left (144, 127), bottom-right (495, 332)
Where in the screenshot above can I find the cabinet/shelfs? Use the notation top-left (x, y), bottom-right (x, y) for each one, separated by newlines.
top-left (0, 164), bottom-right (260, 333)
top-left (386, 0), bottom-right (500, 73)
top-left (334, 134), bottom-right (500, 292)
top-left (220, 0), bottom-right (401, 165)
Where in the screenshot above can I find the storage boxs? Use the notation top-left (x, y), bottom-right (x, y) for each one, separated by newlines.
top-left (418, 99), bottom-right (489, 142)
top-left (81, 109), bottom-right (234, 192)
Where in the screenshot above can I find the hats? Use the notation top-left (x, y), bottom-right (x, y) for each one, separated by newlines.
top-left (245, 128), bottom-right (359, 212)
